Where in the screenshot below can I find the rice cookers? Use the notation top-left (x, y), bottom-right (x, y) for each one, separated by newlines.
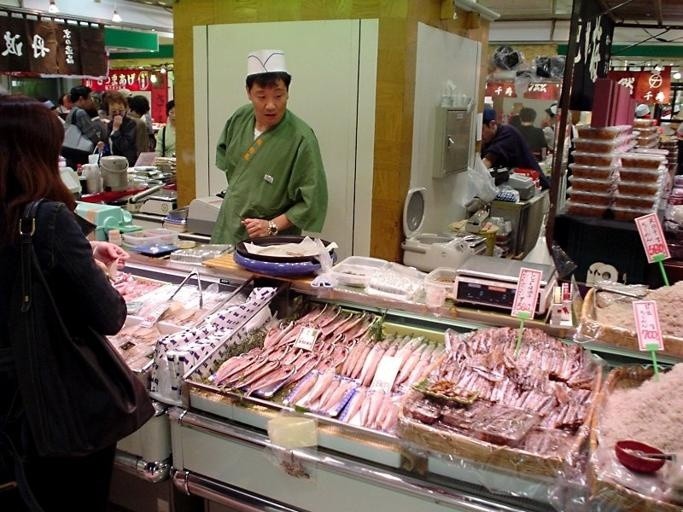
top-left (401, 187), bottom-right (470, 271)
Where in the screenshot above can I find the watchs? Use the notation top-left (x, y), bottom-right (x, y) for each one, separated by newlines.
top-left (268, 220), bottom-right (279, 238)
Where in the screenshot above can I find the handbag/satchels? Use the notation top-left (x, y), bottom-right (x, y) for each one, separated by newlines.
top-left (62, 125), bottom-right (94, 153)
top-left (17, 199), bottom-right (155, 464)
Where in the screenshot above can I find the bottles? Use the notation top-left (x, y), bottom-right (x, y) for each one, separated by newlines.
top-left (77, 164), bottom-right (82, 176)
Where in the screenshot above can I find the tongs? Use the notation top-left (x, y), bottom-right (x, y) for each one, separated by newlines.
top-left (167, 267), bottom-right (203, 308)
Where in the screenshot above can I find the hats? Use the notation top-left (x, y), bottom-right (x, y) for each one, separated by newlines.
top-left (117, 89), bottom-right (133, 98)
top-left (635, 104), bottom-right (650, 117)
top-left (483, 109), bottom-right (495, 124)
top-left (247, 50), bottom-right (286, 75)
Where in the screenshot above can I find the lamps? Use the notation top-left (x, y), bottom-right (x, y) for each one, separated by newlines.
top-left (440, 0), bottom-right (457, 21)
top-left (47, 0), bottom-right (59, 15)
top-left (112, 0), bottom-right (122, 23)
top-left (464, 10), bottom-right (481, 29)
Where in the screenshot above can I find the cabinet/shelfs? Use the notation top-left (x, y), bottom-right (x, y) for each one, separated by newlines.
top-left (67, 229), bottom-right (291, 484)
top-left (168, 261), bottom-right (683, 511)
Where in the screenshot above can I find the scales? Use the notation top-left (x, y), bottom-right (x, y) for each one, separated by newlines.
top-left (451, 255), bottom-right (560, 317)
top-left (138, 190), bottom-right (176, 216)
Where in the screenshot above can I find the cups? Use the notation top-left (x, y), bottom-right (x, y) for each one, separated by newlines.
top-left (79, 176), bottom-right (88, 195)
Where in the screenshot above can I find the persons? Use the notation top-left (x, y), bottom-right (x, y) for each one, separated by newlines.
top-left (474, 93), bottom-right (578, 199)
top-left (43, 79), bottom-right (177, 186)
top-left (2, 91), bottom-right (128, 508)
top-left (209, 49), bottom-right (327, 246)
top-left (634, 103), bottom-right (683, 172)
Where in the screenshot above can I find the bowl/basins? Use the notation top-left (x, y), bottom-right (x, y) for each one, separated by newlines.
top-left (615, 440), bottom-right (666, 473)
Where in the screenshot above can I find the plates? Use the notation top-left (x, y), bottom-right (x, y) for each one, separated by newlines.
top-left (129, 243), bottom-right (178, 256)
top-left (233, 236), bottom-right (337, 276)
